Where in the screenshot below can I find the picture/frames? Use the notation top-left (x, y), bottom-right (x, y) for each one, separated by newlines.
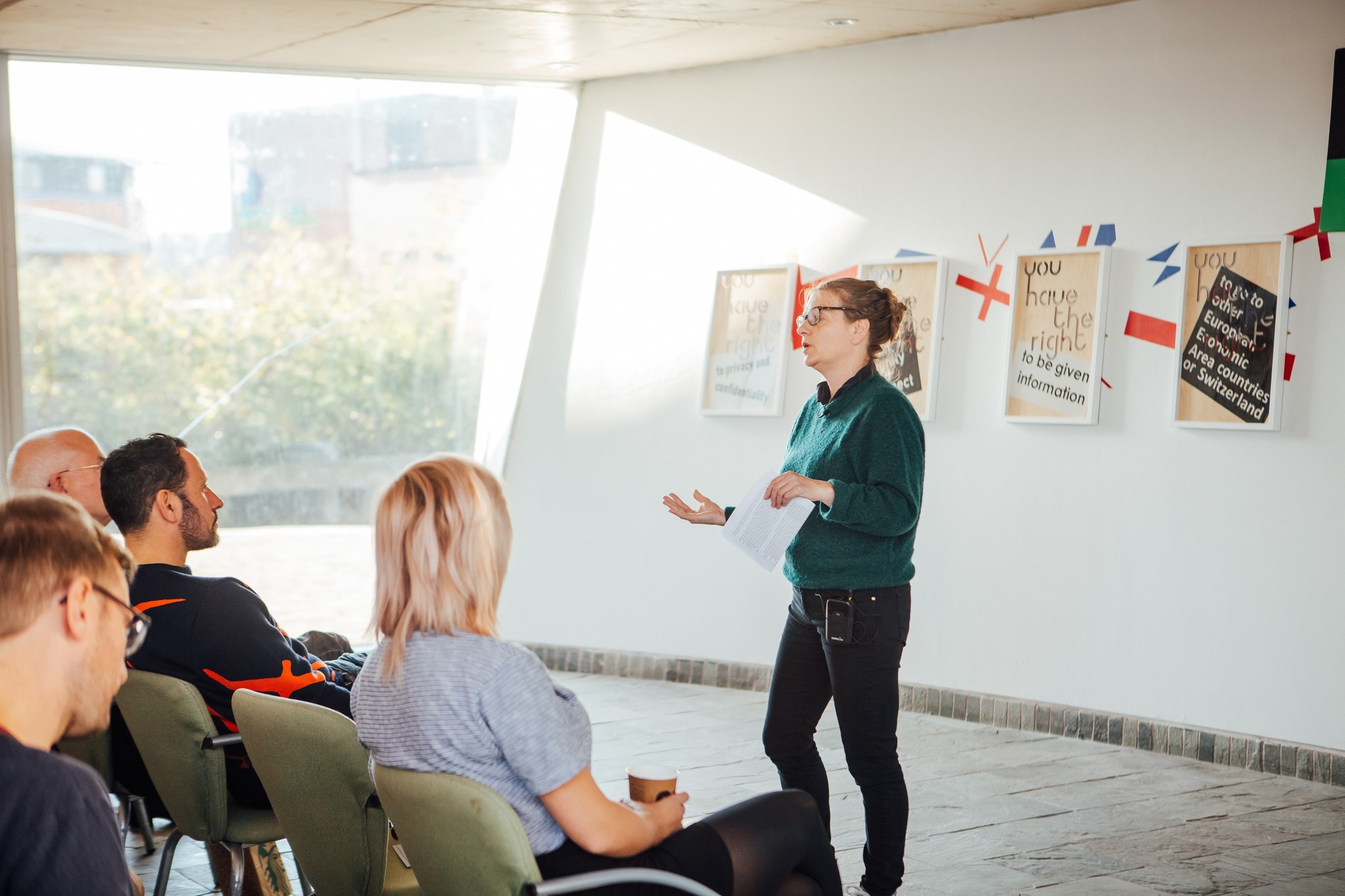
top-left (1001, 244), bottom-right (1102, 429)
top-left (692, 264), bottom-right (798, 420)
top-left (1170, 235), bottom-right (1296, 430)
top-left (858, 256), bottom-right (945, 423)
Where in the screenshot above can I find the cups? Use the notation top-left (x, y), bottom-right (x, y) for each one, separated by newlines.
top-left (624, 764), bottom-right (682, 804)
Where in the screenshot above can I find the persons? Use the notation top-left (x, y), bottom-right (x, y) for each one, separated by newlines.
top-left (663, 279), bottom-right (925, 896)
top-left (349, 456), bottom-right (842, 896)
top-left (0, 429), bottom-right (369, 896)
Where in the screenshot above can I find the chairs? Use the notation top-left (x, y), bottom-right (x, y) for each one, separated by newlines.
top-left (55, 725), bottom-right (154, 853)
top-left (232, 687), bottom-right (418, 895)
top-left (372, 762), bottom-right (722, 895)
top-left (116, 670), bottom-right (314, 895)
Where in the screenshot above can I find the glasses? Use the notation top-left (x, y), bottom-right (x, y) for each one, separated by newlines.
top-left (795, 306), bottom-right (856, 328)
top-left (59, 585), bottom-right (153, 656)
